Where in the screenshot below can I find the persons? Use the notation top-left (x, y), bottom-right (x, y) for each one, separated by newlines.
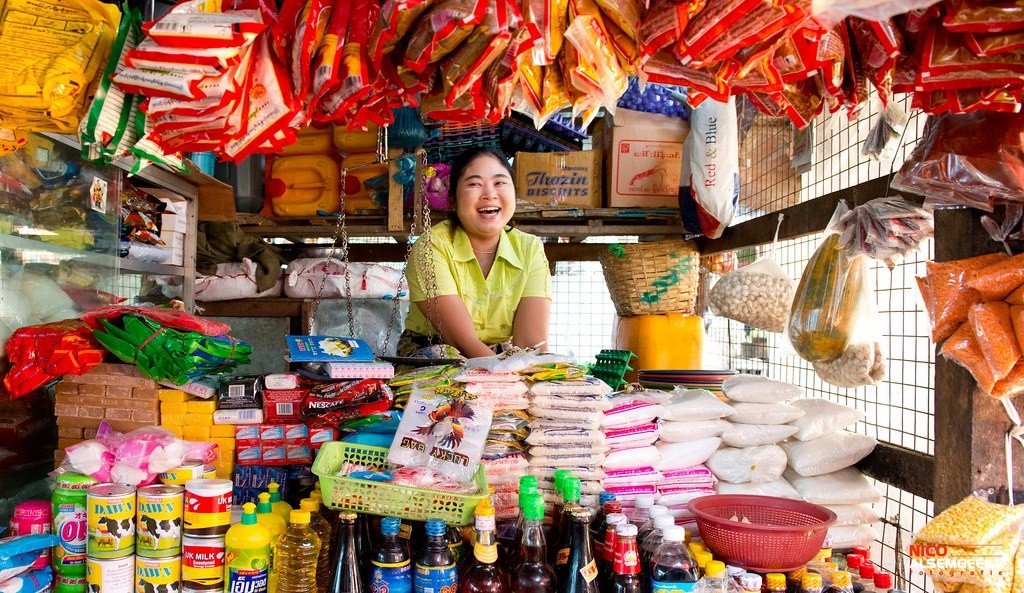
top-left (396, 144), bottom-right (554, 359)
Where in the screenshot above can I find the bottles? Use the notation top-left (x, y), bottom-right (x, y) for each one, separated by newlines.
top-left (546, 470), bottom-right (581, 593)
top-left (599, 513), bottom-right (641, 593)
top-left (629, 494), bottom-right (654, 532)
top-left (558, 503), bottom-right (599, 593)
top-left (507, 475), bottom-right (558, 593)
top-left (444, 524), bottom-right (465, 586)
top-left (268, 482), bottom-right (293, 528)
top-left (300, 498), bottom-right (332, 593)
top-left (331, 510), bottom-right (375, 593)
top-left (368, 516), bottom-right (457, 593)
top-left (310, 489), bottom-right (340, 585)
top-left (727, 564), bottom-right (786, 593)
top-left (273, 509), bottom-right (322, 593)
top-left (788, 544), bottom-right (903, 593)
top-left (315, 481), bottom-right (321, 489)
top-left (639, 515), bottom-right (726, 593)
top-left (589, 491), bottom-right (622, 593)
top-left (636, 505), bottom-right (669, 551)
top-left (458, 485), bottom-right (510, 593)
top-left (224, 493), bottom-right (286, 593)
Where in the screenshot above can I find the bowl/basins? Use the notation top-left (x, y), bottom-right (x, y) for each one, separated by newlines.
top-left (302, 244), bottom-right (345, 260)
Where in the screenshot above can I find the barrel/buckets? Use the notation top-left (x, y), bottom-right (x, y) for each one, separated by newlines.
top-left (190, 152), bottom-right (264, 215)
top-left (268, 120), bottom-right (409, 216)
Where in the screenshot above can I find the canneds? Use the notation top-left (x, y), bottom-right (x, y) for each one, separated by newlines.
top-left (86, 459), bottom-right (234, 593)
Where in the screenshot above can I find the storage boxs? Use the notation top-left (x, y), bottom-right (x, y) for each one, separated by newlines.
top-left (512, 107), bottom-right (690, 208)
top-left (311, 440), bottom-right (491, 526)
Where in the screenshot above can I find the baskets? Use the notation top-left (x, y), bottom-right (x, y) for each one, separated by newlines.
top-left (687, 493), bottom-right (839, 573)
top-left (311, 440), bottom-right (490, 526)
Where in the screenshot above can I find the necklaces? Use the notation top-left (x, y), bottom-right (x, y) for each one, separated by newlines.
top-left (473, 248), bottom-right (497, 254)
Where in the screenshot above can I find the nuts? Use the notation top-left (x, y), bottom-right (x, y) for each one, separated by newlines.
top-left (812, 343), bottom-right (885, 387)
top-left (709, 272), bottom-right (793, 332)
